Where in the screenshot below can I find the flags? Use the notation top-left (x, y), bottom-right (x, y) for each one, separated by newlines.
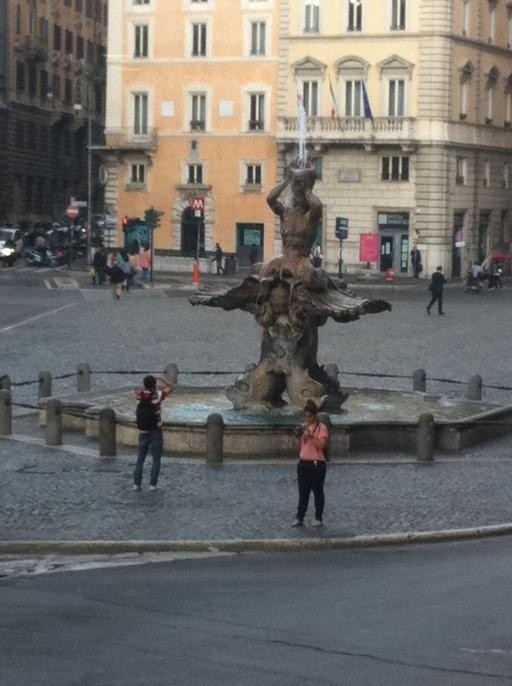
top-left (362, 80), bottom-right (375, 128)
top-left (330, 81), bottom-right (338, 122)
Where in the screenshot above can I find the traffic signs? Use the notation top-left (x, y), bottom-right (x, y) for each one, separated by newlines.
top-left (71, 201), bottom-right (88, 208)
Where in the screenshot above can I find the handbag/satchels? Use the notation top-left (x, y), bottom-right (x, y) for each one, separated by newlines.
top-left (428, 282), bottom-right (444, 295)
top-left (416, 263), bottom-right (423, 272)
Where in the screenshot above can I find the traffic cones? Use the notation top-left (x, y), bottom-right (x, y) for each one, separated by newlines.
top-left (191, 263), bottom-right (200, 287)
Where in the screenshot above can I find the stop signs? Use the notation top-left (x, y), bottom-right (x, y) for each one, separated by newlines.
top-left (67, 206), bottom-right (80, 218)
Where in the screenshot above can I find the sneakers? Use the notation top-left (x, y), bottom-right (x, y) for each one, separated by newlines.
top-left (132, 484), bottom-right (143, 491)
top-left (149, 483), bottom-right (162, 491)
top-left (426, 306), bottom-right (445, 316)
top-left (311, 519), bottom-right (323, 528)
top-left (289, 519), bottom-right (303, 527)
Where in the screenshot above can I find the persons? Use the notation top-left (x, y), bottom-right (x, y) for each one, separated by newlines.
top-left (212, 243), bottom-right (226, 276)
top-left (250, 154), bottom-right (347, 293)
top-left (249, 243), bottom-right (258, 265)
top-left (291, 399), bottom-right (329, 527)
top-left (411, 246), bottom-right (422, 278)
top-left (313, 241), bottom-right (321, 258)
top-left (133, 375), bottom-right (175, 491)
top-left (89, 238), bottom-right (151, 300)
top-left (426, 266), bottom-right (446, 315)
top-left (467, 261), bottom-right (506, 293)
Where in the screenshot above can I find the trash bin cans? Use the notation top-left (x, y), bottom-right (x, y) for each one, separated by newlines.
top-left (225, 258), bottom-right (237, 275)
top-left (90, 246), bottom-right (100, 264)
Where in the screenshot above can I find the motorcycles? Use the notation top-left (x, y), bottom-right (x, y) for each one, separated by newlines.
top-left (23, 232), bottom-right (101, 270)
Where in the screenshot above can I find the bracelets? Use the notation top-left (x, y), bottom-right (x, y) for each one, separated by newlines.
top-left (309, 435), bottom-right (313, 441)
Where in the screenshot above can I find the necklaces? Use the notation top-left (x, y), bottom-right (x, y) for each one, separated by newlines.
top-left (307, 422), bottom-right (319, 435)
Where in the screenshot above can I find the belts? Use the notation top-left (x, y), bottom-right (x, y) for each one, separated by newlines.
top-left (299, 459), bottom-right (326, 465)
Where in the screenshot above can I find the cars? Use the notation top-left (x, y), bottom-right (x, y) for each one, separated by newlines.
top-left (0, 237), bottom-right (16, 266)
top-left (15, 212), bottom-right (106, 243)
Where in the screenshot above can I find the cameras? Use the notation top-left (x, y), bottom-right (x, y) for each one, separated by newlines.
top-left (293, 424), bottom-right (303, 438)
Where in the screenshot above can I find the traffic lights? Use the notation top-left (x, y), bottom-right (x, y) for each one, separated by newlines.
top-left (122, 214), bottom-right (133, 236)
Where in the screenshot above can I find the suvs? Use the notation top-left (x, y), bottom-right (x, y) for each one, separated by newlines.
top-left (0, 226), bottom-right (25, 257)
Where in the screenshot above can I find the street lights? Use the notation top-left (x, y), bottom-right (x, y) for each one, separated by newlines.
top-left (74, 101), bottom-right (95, 265)
top-left (46, 91), bottom-right (74, 212)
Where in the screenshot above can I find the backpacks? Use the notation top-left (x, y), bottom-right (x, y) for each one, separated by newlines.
top-left (134, 389), bottom-right (163, 432)
